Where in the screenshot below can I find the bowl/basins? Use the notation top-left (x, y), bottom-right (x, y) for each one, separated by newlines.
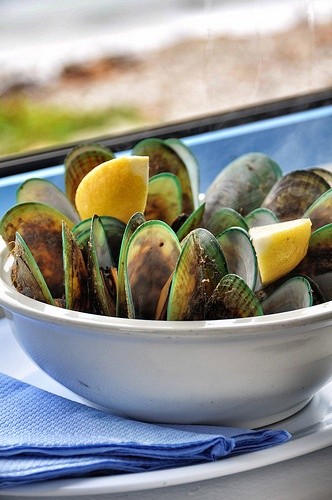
top-left (1, 237), bottom-right (332, 430)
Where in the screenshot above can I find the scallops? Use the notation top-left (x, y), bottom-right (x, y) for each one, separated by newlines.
top-left (0, 135), bottom-right (332, 321)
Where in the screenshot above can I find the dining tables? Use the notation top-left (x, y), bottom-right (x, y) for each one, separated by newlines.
top-left (0, 103), bottom-right (332, 500)
